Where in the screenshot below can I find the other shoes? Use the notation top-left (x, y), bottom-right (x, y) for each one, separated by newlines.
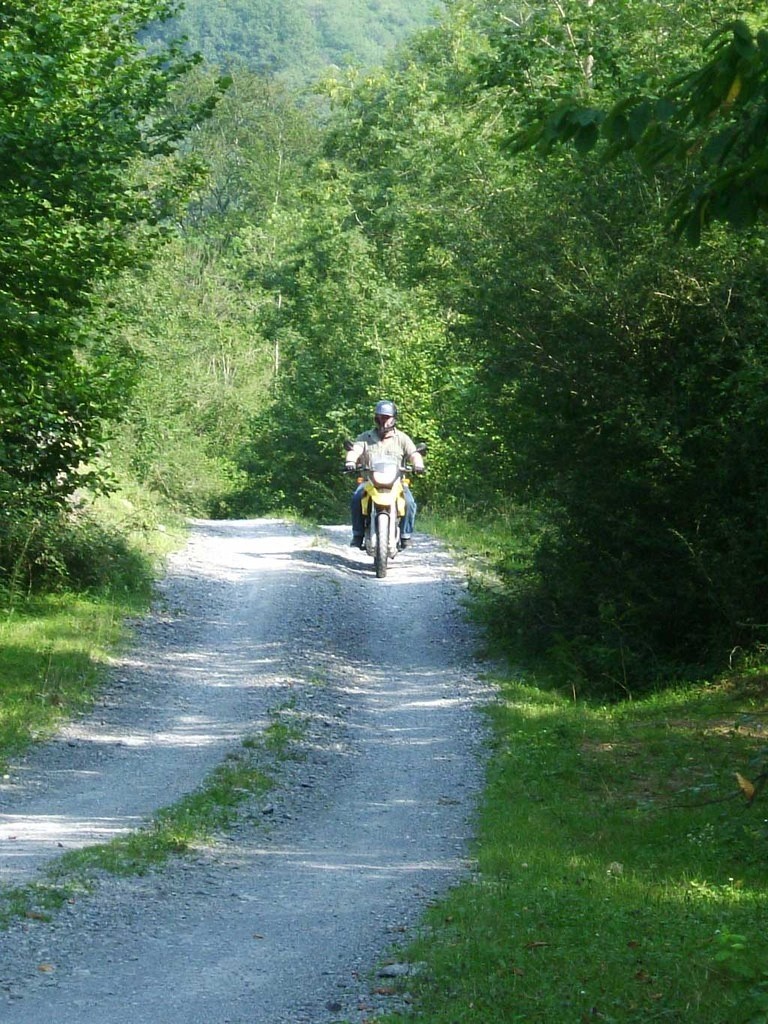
top-left (401, 538), bottom-right (412, 549)
top-left (350, 534), bottom-right (363, 547)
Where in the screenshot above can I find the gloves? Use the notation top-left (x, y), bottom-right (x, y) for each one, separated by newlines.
top-left (412, 465), bottom-right (423, 475)
top-left (344, 461), bottom-right (356, 471)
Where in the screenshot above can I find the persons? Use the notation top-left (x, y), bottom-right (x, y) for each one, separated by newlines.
top-left (346, 401), bottom-right (424, 547)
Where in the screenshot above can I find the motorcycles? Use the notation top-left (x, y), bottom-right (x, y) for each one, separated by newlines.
top-left (343, 440), bottom-right (428, 578)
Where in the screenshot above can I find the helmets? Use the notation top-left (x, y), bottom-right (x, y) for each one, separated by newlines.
top-left (374, 400), bottom-right (397, 423)
